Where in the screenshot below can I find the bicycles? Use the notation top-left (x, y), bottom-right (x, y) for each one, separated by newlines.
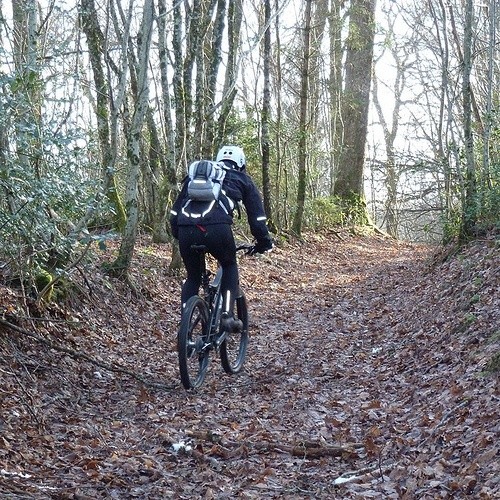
top-left (176, 240), bottom-right (280, 391)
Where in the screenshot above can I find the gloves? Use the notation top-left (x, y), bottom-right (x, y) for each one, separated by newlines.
top-left (254, 238), bottom-right (272, 253)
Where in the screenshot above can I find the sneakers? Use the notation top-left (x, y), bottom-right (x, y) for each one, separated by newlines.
top-left (219, 314), bottom-right (244, 333)
top-left (186, 342), bottom-right (196, 360)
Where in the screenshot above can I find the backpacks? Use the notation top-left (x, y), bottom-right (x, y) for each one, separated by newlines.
top-left (188, 160), bottom-right (227, 200)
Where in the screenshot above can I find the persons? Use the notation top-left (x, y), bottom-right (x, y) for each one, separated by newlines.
top-left (169, 145), bottom-right (272, 357)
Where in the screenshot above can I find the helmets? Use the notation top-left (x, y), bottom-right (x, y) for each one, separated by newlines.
top-left (216, 146), bottom-right (245, 168)
